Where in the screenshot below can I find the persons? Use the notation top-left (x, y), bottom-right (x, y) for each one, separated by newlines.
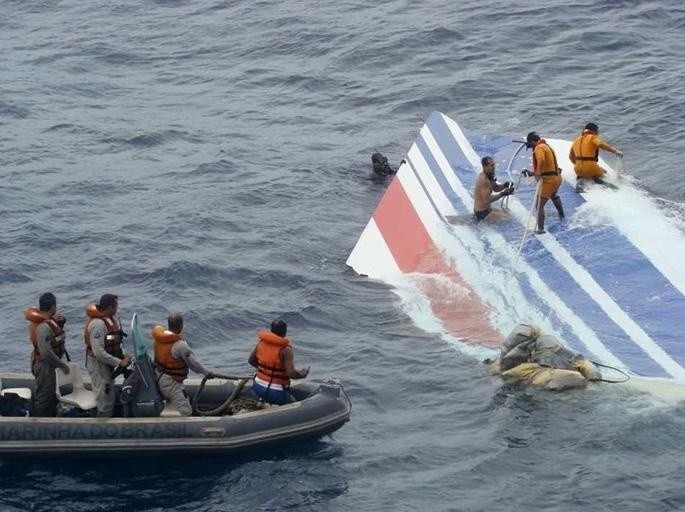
top-left (473, 155), bottom-right (515, 224)
top-left (371, 152), bottom-right (406, 178)
top-left (153, 313), bottom-right (215, 416)
top-left (569, 121), bottom-right (625, 191)
top-left (28, 291), bottom-right (71, 416)
top-left (83, 293), bottom-right (133, 416)
top-left (522, 130), bottom-right (566, 235)
top-left (248, 318), bottom-right (312, 405)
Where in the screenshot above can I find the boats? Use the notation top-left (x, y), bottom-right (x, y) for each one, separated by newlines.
top-left (0, 372), bottom-right (351, 460)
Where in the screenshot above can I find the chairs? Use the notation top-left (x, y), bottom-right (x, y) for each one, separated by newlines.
top-left (54, 362), bottom-right (97, 416)
